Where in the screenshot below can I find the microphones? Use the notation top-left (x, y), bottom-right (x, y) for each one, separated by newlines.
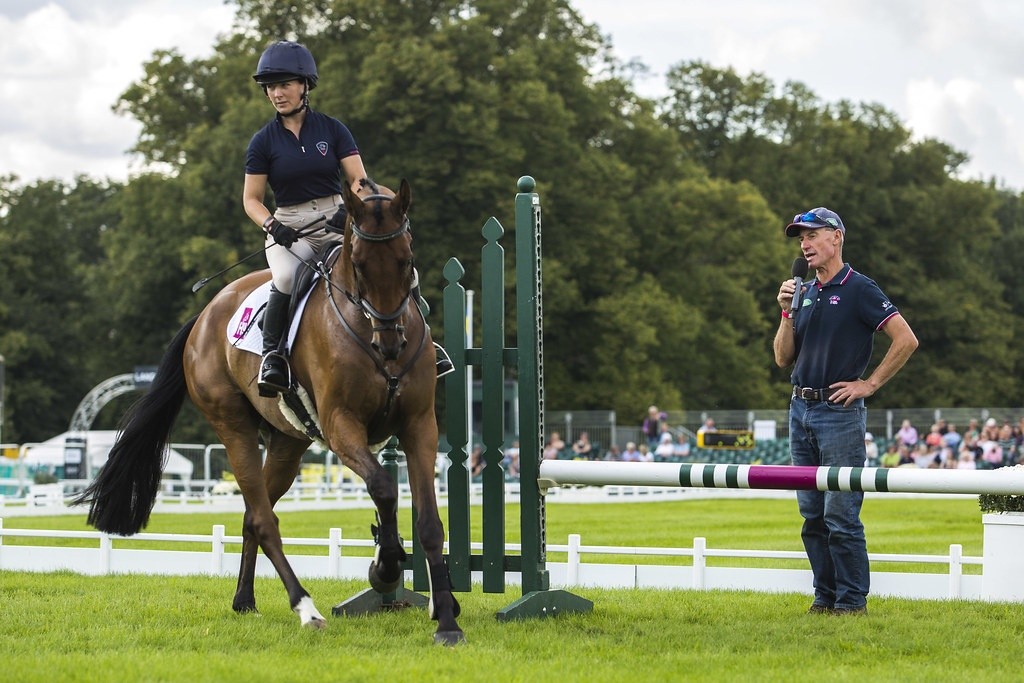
top-left (790, 257), bottom-right (808, 311)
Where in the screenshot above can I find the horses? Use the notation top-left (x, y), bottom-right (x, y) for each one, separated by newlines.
top-left (61, 177), bottom-right (468, 648)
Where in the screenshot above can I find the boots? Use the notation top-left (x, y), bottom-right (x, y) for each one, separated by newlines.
top-left (258, 282), bottom-right (292, 398)
top-left (411, 286), bottom-right (451, 375)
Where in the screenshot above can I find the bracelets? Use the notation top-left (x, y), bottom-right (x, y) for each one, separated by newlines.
top-left (261, 216), bottom-right (276, 240)
top-left (782, 310), bottom-right (792, 319)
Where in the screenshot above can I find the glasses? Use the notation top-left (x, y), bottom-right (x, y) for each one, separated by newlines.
top-left (793, 213), bottom-right (836, 231)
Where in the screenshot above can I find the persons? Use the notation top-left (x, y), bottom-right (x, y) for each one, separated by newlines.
top-left (643, 405), bottom-right (667, 442)
top-left (621, 441), bottom-right (639, 462)
top-left (865, 432), bottom-right (878, 467)
top-left (470, 430), bottom-right (691, 479)
top-left (893, 418), bottom-right (1024, 469)
top-left (773, 206), bottom-right (919, 615)
top-left (637, 444), bottom-right (654, 462)
top-left (879, 443), bottom-right (900, 468)
top-left (698, 418), bottom-right (716, 431)
top-left (242, 41), bottom-right (452, 397)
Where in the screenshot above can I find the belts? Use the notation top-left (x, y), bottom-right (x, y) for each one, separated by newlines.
top-left (792, 384), bottom-right (845, 400)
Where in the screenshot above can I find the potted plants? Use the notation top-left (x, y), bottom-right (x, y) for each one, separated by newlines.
top-left (977, 493), bottom-right (1024, 605)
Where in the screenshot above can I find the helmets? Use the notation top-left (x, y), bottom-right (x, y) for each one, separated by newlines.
top-left (252, 40), bottom-right (319, 91)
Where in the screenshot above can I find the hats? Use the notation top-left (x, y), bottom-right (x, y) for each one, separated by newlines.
top-left (865, 432), bottom-right (873, 441)
top-left (786, 207), bottom-right (845, 237)
top-left (966, 444), bottom-right (983, 458)
top-left (986, 417), bottom-right (996, 427)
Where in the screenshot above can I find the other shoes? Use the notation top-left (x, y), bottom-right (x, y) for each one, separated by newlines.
top-left (808, 605), bottom-right (867, 616)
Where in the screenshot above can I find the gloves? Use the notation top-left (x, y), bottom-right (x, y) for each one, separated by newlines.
top-left (326, 204), bottom-right (347, 236)
top-left (269, 222), bottom-right (298, 249)
